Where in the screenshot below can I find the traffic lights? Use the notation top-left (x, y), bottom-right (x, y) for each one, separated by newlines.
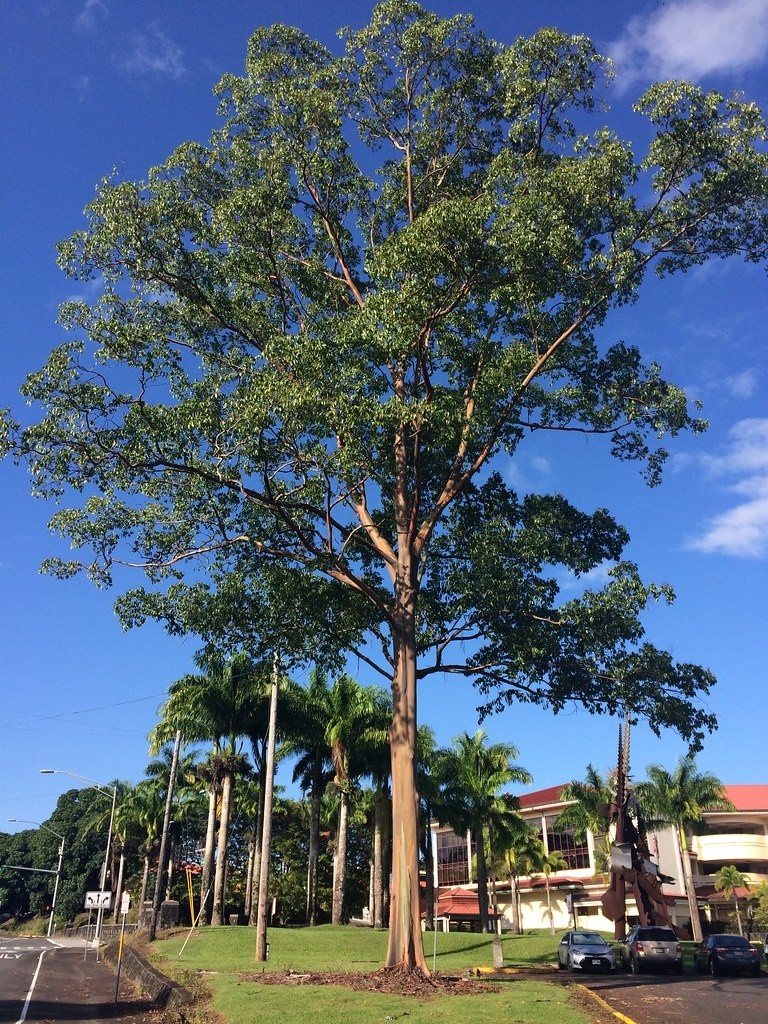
top-left (45, 905), bottom-right (54, 911)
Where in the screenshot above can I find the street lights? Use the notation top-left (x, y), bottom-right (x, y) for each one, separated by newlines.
top-left (39, 770), bottom-right (117, 947)
top-left (8, 818), bottom-right (66, 936)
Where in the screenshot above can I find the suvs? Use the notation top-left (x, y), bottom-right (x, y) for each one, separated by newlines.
top-left (619, 927), bottom-right (684, 974)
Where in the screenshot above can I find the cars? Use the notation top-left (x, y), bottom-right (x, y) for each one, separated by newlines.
top-left (695, 934), bottom-right (760, 975)
top-left (558, 931), bottom-right (616, 972)
